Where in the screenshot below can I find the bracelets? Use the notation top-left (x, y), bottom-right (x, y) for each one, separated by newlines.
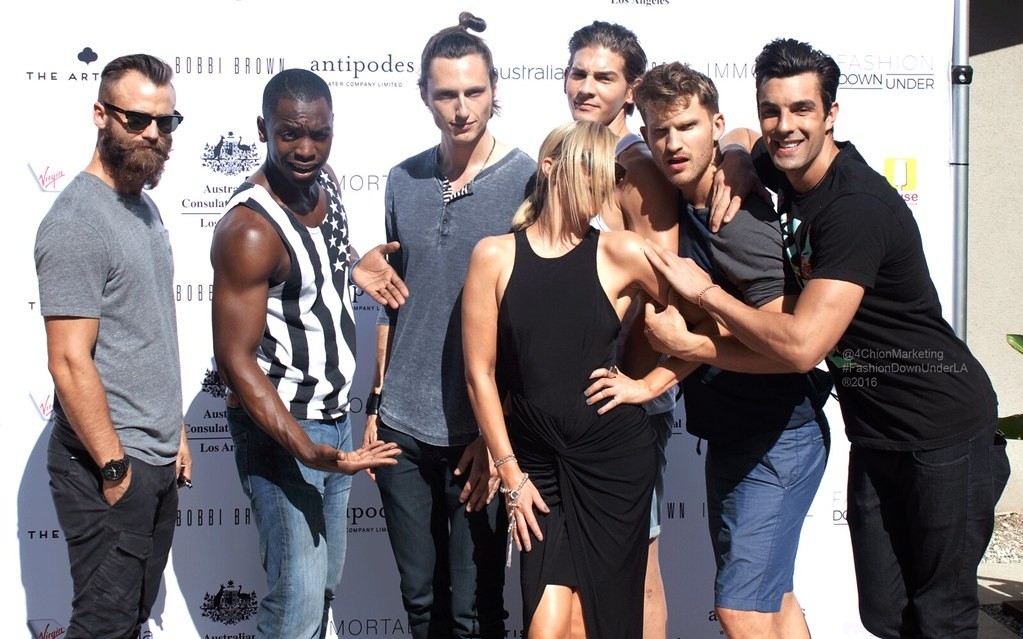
top-left (351, 257), bottom-right (361, 284)
top-left (492, 453), bottom-right (520, 467)
top-left (364, 390), bottom-right (381, 416)
top-left (504, 414), bottom-right (512, 427)
top-left (499, 471), bottom-right (529, 567)
top-left (698, 284), bottom-right (717, 309)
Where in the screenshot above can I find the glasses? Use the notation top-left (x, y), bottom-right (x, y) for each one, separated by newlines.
top-left (583, 150), bottom-right (628, 186)
top-left (104, 103), bottom-right (184, 134)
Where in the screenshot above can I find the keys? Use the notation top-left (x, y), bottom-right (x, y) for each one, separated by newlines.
top-left (177, 464), bottom-right (193, 489)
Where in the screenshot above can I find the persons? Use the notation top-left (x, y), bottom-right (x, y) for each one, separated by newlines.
top-left (33, 54), bottom-right (194, 639)
top-left (630, 62), bottom-right (834, 639)
top-left (540, 17), bottom-right (675, 639)
top-left (461, 118), bottom-right (726, 639)
top-left (208, 69), bottom-right (409, 639)
top-left (645, 38), bottom-right (1011, 639)
top-left (360, 10), bottom-right (539, 639)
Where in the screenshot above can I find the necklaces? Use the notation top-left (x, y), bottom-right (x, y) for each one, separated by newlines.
top-left (473, 134), bottom-right (497, 184)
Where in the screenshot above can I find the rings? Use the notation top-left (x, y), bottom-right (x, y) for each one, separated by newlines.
top-left (601, 391), bottom-right (606, 398)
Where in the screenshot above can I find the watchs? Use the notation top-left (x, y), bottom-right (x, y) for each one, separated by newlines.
top-left (99, 453), bottom-right (129, 481)
